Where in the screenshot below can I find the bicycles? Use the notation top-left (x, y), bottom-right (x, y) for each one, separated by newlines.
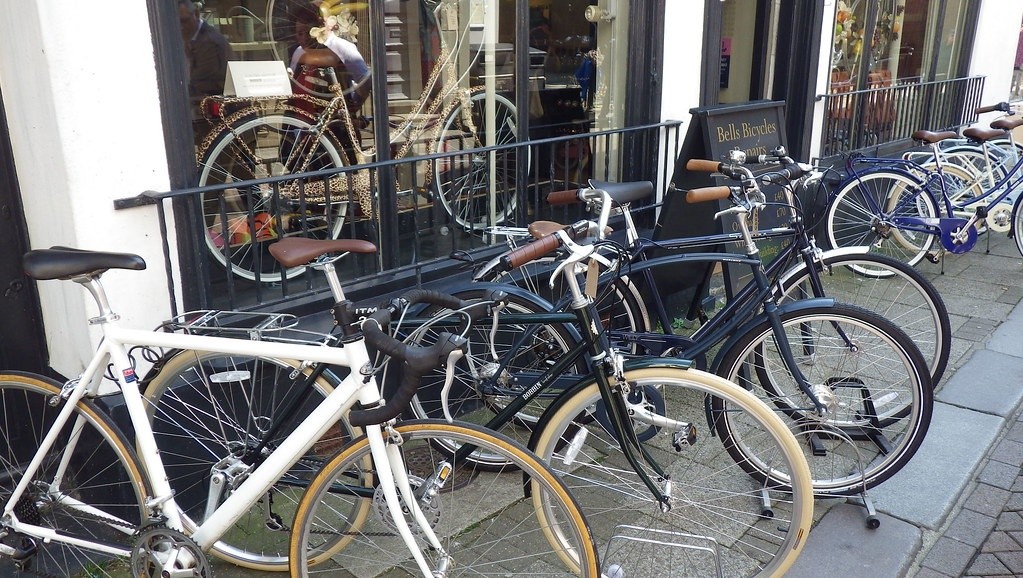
top-left (1, 147), bottom-right (954, 578)
top-left (821, 102), bottom-right (1022, 280)
top-left (194, 1), bottom-right (535, 288)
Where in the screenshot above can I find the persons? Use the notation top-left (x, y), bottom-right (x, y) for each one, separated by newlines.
top-left (179, 3), bottom-right (272, 249)
top-left (282, 4), bottom-right (364, 225)
